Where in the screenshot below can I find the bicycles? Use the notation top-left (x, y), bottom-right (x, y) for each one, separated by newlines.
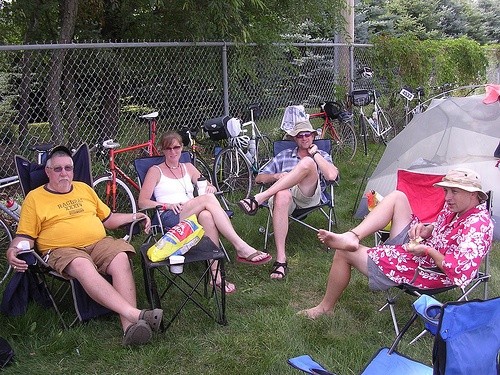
top-left (280, 98), bottom-right (358, 167)
top-left (430, 82), bottom-right (456, 99)
top-left (397, 86), bottom-right (429, 127)
top-left (346, 89), bottom-right (397, 155)
top-left (199, 103), bottom-right (277, 207)
top-left (87, 111), bottom-right (214, 230)
top-left (0, 143), bottom-right (55, 284)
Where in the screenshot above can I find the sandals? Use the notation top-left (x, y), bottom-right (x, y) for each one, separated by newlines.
top-left (269, 261), bottom-right (288, 280)
top-left (237, 196), bottom-right (259, 216)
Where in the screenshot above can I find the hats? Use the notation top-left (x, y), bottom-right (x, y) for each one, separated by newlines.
top-left (44, 145), bottom-right (72, 168)
top-left (432, 169), bottom-right (489, 202)
top-left (288, 120), bottom-right (319, 137)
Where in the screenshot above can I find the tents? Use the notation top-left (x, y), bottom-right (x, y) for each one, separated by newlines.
top-left (351, 84), bottom-right (500, 241)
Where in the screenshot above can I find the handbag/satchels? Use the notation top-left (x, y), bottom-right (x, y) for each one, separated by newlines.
top-left (351, 89), bottom-right (372, 107)
top-left (320, 100), bottom-right (343, 120)
top-left (147, 214), bottom-right (206, 263)
top-left (203, 115), bottom-right (231, 141)
top-left (364, 189), bottom-right (391, 231)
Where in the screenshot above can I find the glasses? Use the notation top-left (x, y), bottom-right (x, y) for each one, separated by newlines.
top-left (46, 165), bottom-right (74, 172)
top-left (295, 132), bottom-right (314, 138)
top-left (161, 145), bottom-right (182, 152)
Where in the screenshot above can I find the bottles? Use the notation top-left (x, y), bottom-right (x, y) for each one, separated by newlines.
top-left (367, 116), bottom-right (377, 129)
top-left (249, 137), bottom-right (256, 157)
top-left (315, 126), bottom-right (322, 140)
top-left (5, 199), bottom-right (22, 218)
top-left (372, 109), bottom-right (377, 123)
top-left (196, 174), bottom-right (207, 195)
top-left (243, 148), bottom-right (255, 164)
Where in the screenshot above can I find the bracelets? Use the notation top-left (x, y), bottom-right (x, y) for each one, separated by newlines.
top-left (312, 151), bottom-right (320, 157)
top-left (133, 213), bottom-right (136, 220)
top-left (163, 203), bottom-right (167, 211)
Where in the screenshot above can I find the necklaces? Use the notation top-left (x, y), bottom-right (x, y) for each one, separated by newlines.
top-left (165, 160), bottom-right (188, 196)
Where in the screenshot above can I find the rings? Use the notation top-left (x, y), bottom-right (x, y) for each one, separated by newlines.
top-left (14, 266), bottom-right (17, 269)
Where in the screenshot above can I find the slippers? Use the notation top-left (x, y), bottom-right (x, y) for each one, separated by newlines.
top-left (121, 319), bottom-right (152, 345)
top-left (209, 279), bottom-right (236, 294)
top-left (138, 308), bottom-right (163, 332)
top-left (237, 250), bottom-right (273, 265)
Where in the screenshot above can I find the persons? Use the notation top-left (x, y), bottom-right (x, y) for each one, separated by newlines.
top-left (239, 119), bottom-right (338, 278)
top-left (6, 145), bottom-right (162, 345)
top-left (139, 132), bottom-right (272, 293)
top-left (296, 169), bottom-right (494, 317)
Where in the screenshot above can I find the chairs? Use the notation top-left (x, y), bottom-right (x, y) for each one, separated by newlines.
top-left (378, 189), bottom-right (494, 345)
top-left (287, 292), bottom-right (500, 375)
top-left (252, 138), bottom-right (340, 255)
top-left (364, 169), bottom-right (446, 247)
top-left (13, 142), bottom-right (146, 331)
top-left (134, 151), bottom-right (235, 263)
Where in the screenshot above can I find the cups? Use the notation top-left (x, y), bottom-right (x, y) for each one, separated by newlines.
top-left (169, 255), bottom-right (185, 274)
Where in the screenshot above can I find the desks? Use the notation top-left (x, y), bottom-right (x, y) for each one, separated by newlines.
top-left (140, 235), bottom-right (227, 333)
